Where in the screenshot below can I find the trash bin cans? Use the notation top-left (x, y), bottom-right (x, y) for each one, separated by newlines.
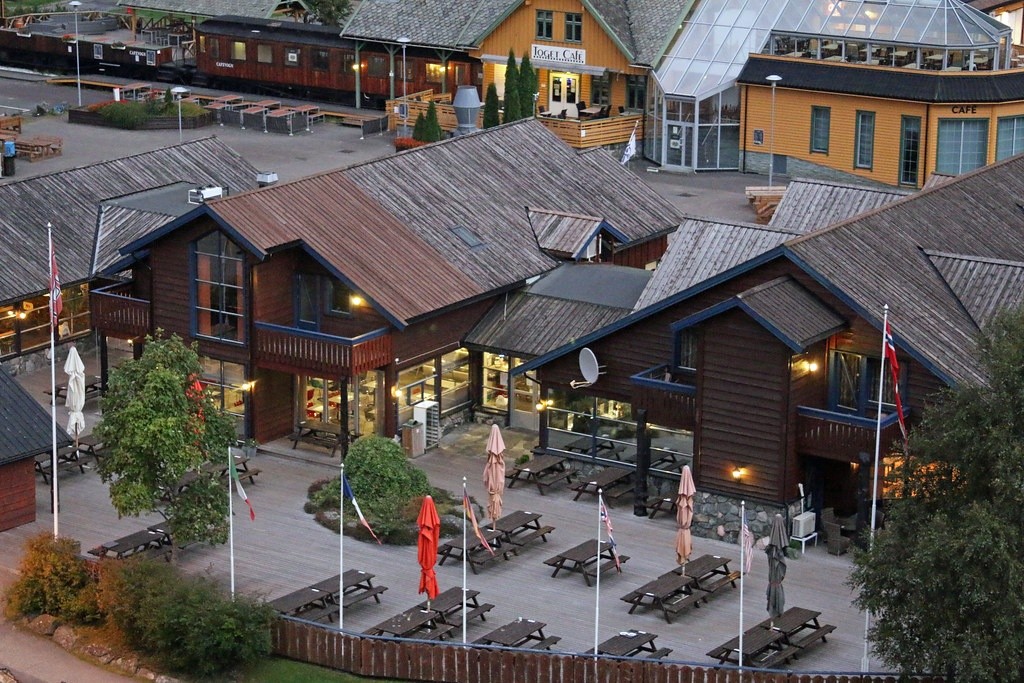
top-left (402, 419), bottom-right (424, 459)
top-left (3, 155), bottom-right (16, 176)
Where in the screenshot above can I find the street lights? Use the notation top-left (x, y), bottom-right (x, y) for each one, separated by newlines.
top-left (171, 87), bottom-right (189, 143)
top-left (396, 37), bottom-right (411, 137)
top-left (68, 1), bottom-right (83, 107)
top-left (765, 74), bottom-right (783, 193)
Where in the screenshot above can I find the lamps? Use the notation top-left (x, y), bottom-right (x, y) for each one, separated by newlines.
top-left (809, 359), bottom-right (817, 373)
top-left (732, 465), bottom-right (741, 486)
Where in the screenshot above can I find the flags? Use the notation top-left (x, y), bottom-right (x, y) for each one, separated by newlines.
top-left (744, 513), bottom-right (754, 574)
top-left (49, 240), bottom-right (63, 327)
top-left (600, 499), bottom-right (621, 572)
top-left (230, 455), bottom-right (255, 520)
top-left (885, 322), bottom-right (908, 444)
top-left (464, 490), bottom-right (495, 555)
top-left (343, 474), bottom-right (381, 545)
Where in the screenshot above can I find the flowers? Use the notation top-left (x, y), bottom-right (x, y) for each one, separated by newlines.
top-left (243, 438), bottom-right (261, 449)
top-left (112, 41), bottom-right (127, 48)
top-left (62, 34), bottom-right (76, 42)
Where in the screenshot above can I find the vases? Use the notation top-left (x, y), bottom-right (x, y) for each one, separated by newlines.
top-left (242, 446), bottom-right (257, 458)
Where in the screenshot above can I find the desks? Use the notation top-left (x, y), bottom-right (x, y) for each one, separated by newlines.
top-left (291, 419), bottom-right (342, 458)
top-left (626, 445), bottom-right (683, 473)
top-left (34, 374), bottom-right (255, 563)
top-left (0, 29), bottom-right (989, 163)
top-left (507, 454), bottom-right (679, 519)
top-left (306, 377), bottom-right (401, 421)
top-left (837, 507), bottom-right (866, 531)
top-left (402, 422), bottom-right (423, 459)
top-left (564, 436), bottom-right (621, 461)
top-left (227, 404), bottom-right (244, 415)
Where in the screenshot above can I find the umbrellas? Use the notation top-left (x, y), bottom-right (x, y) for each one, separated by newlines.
top-left (416, 495), bottom-right (440, 633)
top-left (764, 515), bottom-right (789, 654)
top-left (63, 346), bottom-right (86, 457)
top-left (675, 465), bottom-right (696, 598)
top-left (482, 424), bottom-right (506, 552)
top-left (185, 373), bottom-right (206, 447)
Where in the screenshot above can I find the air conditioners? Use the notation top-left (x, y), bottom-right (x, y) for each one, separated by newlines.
top-left (792, 511), bottom-right (816, 539)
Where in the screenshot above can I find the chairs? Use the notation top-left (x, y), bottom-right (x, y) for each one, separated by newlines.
top-left (876, 510), bottom-right (885, 528)
top-left (823, 520), bottom-right (851, 558)
top-left (494, 385), bottom-right (505, 406)
top-left (821, 507), bottom-right (837, 543)
top-left (452, 370), bottom-right (468, 386)
top-left (422, 365), bottom-right (434, 379)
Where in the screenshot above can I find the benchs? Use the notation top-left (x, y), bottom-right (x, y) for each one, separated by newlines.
top-left (43, 456), bottom-right (837, 668)
top-left (660, 458), bottom-right (691, 471)
top-left (85, 391), bottom-right (102, 401)
top-left (599, 445), bottom-right (627, 458)
top-left (287, 433), bottom-right (337, 450)
top-left (349, 431), bottom-right (364, 443)
top-left (43, 389), bottom-right (52, 396)
